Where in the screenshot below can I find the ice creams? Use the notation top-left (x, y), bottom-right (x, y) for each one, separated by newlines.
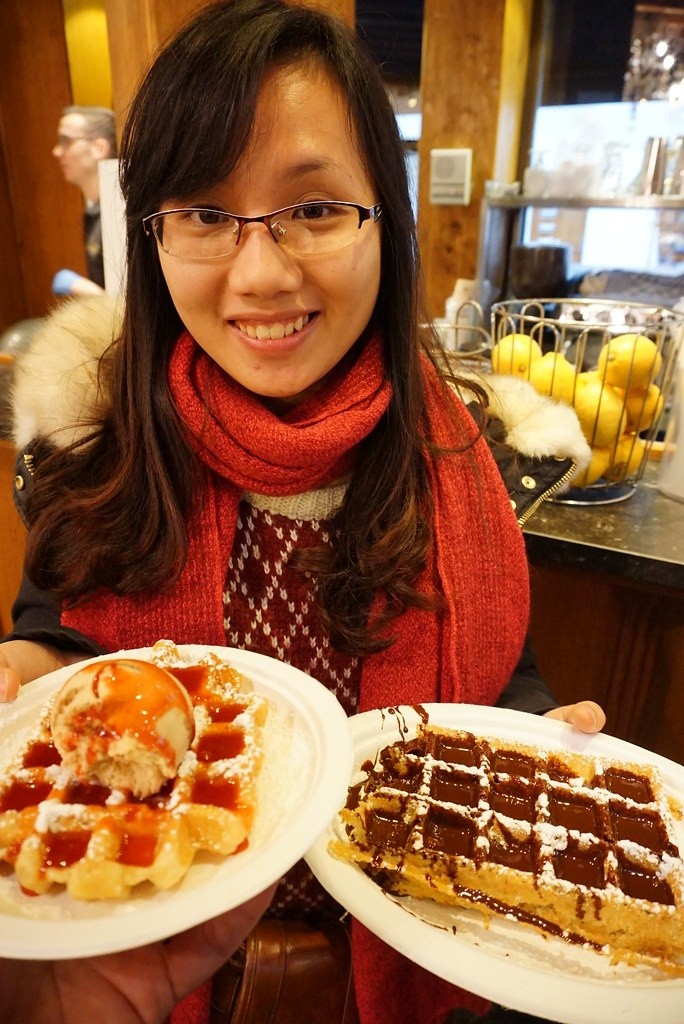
top-left (50, 657), bottom-right (196, 798)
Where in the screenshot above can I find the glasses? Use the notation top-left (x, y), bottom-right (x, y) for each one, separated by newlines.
top-left (58, 133), bottom-right (90, 151)
top-left (142, 201), bottom-right (382, 259)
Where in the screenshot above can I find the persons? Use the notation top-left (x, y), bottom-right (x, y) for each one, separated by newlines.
top-left (0, 0), bottom-right (607, 1024)
top-left (52, 106), bottom-right (118, 291)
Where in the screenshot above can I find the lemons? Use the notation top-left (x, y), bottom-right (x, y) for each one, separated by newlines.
top-left (493, 334), bottom-right (663, 486)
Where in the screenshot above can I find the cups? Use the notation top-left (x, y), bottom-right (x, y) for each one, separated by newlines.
top-left (646, 137), bottom-right (684, 194)
top-left (510, 247), bottom-right (565, 337)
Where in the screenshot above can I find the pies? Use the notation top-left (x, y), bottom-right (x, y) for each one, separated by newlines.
top-left (336, 730), bottom-right (684, 953)
top-left (1, 641), bottom-right (270, 899)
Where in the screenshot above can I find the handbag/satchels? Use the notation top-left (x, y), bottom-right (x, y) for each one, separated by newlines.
top-left (212, 918), bottom-right (350, 1024)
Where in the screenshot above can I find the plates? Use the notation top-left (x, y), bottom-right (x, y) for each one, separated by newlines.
top-left (0, 643), bottom-right (352, 960)
top-left (303, 702), bottom-right (684, 1024)
